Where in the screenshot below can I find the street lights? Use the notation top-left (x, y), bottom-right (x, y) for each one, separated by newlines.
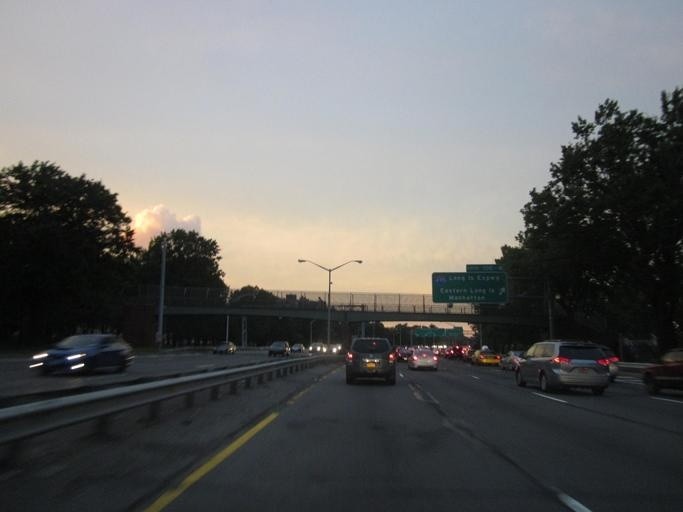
top-left (298, 258), bottom-right (362, 348)
top-left (155, 232), bottom-right (168, 354)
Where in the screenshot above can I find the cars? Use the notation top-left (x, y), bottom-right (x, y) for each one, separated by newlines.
top-left (643, 348), bottom-right (683, 398)
top-left (213, 340), bottom-right (236, 354)
top-left (28, 333), bottom-right (134, 376)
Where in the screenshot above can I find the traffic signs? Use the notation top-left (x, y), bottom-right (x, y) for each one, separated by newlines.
top-left (431, 271), bottom-right (508, 306)
